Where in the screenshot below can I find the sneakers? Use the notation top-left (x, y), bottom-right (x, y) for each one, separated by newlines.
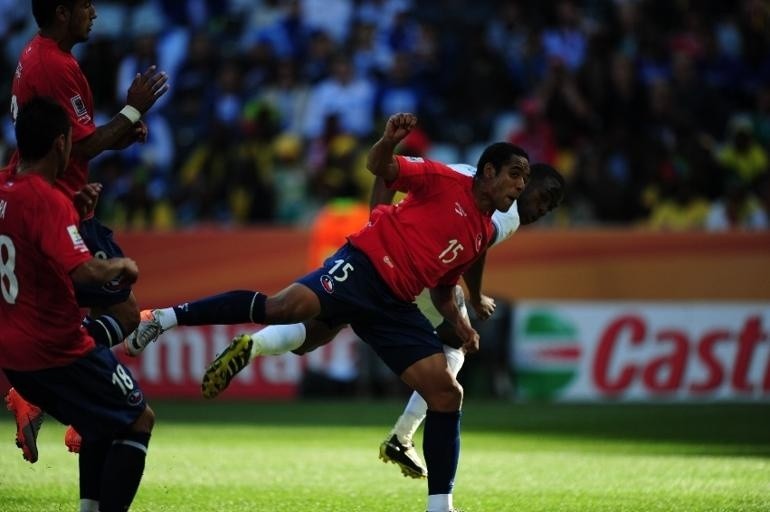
top-left (123, 308), bottom-right (164, 357)
top-left (3, 387), bottom-right (44, 464)
top-left (64, 424), bottom-right (82, 453)
top-left (200, 334), bottom-right (252, 400)
top-left (379, 434), bottom-right (428, 479)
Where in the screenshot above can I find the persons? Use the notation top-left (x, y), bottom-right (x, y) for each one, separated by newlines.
top-left (0, 96), bottom-right (156, 512)
top-left (123, 111), bottom-right (532, 512)
top-left (4, 0), bottom-right (170, 463)
top-left (201, 143), bottom-right (566, 480)
top-left (3, 0), bottom-right (770, 229)
